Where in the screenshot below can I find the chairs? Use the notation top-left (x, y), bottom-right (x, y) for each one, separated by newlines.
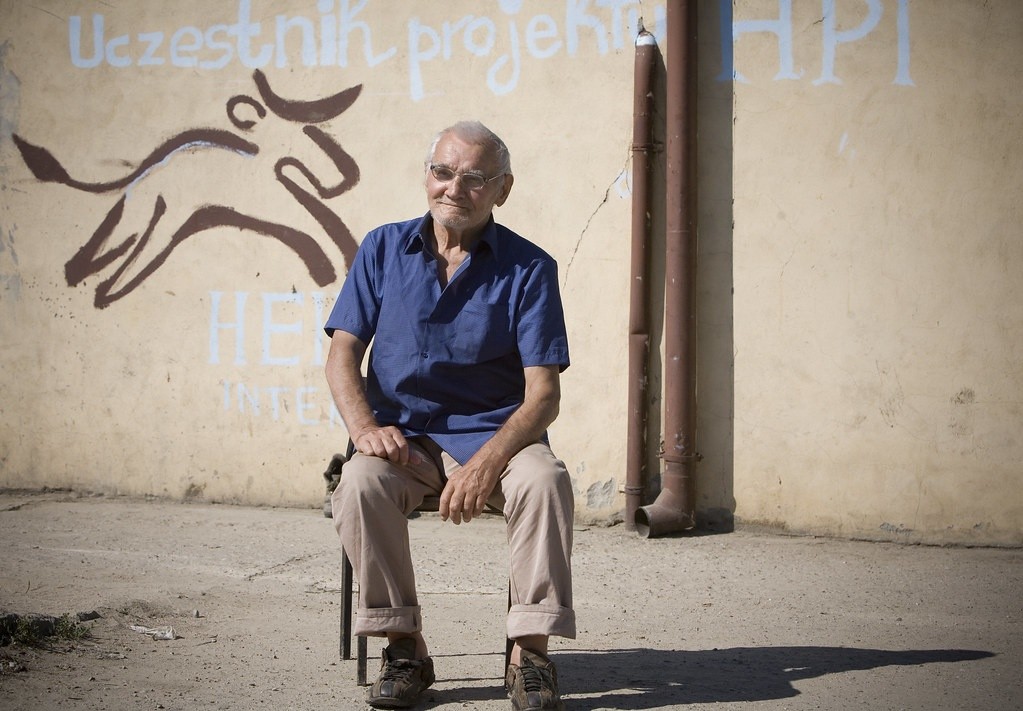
top-left (325, 376), bottom-right (514, 689)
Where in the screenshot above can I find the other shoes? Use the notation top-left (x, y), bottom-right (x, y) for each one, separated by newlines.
top-left (323, 453), bottom-right (347, 518)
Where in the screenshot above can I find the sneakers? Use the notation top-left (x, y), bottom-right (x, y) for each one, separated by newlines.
top-left (505, 647), bottom-right (561, 711)
top-left (364, 637), bottom-right (435, 706)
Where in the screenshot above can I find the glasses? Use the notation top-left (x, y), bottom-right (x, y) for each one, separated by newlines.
top-left (430, 160), bottom-right (507, 190)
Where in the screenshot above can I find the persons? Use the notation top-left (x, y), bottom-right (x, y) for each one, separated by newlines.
top-left (323, 119), bottom-right (576, 711)
